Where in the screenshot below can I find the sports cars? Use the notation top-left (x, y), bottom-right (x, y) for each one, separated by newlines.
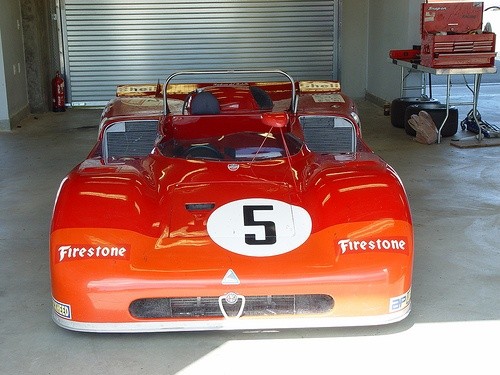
top-left (50, 68), bottom-right (416, 336)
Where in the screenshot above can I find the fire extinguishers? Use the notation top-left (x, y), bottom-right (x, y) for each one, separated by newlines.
top-left (52, 70), bottom-right (67, 112)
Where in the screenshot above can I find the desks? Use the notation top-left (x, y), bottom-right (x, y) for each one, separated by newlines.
top-left (387, 58), bottom-right (498, 144)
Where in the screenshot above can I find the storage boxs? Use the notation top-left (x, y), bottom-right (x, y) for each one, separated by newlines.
top-left (419, 2), bottom-right (497, 68)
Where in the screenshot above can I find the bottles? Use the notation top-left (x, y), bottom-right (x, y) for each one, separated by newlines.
top-left (384, 101), bottom-right (390, 116)
